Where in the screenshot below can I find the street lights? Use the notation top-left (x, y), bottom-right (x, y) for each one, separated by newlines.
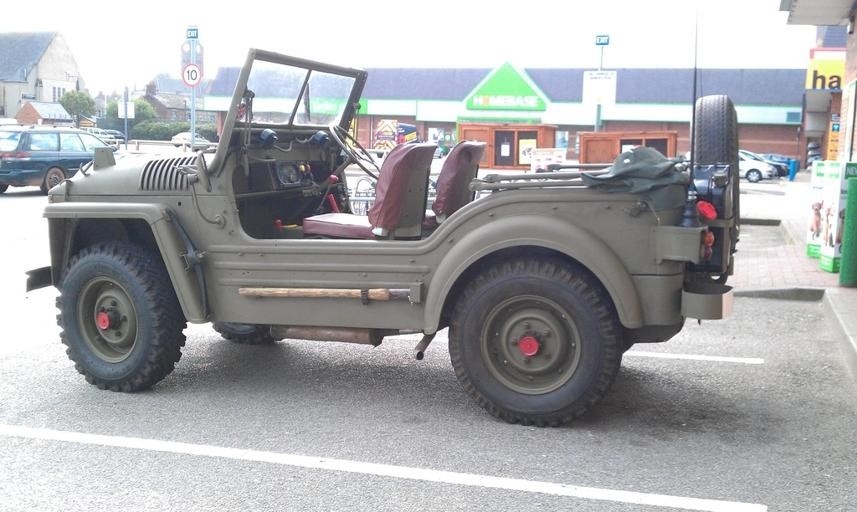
top-left (595, 33), bottom-right (613, 128)
top-left (185, 24), bottom-right (199, 150)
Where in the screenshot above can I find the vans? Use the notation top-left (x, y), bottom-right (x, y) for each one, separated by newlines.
top-left (374, 119), bottom-right (419, 157)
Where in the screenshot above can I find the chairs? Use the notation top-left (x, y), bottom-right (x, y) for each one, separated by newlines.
top-left (300, 139), bottom-right (437, 243)
top-left (425, 138), bottom-right (487, 235)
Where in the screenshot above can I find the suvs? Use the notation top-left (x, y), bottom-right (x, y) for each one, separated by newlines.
top-left (18, 45), bottom-right (745, 426)
top-left (1, 116), bottom-right (125, 196)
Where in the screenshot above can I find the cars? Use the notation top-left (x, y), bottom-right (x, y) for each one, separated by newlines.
top-left (734, 148), bottom-right (800, 183)
top-left (171, 131), bottom-right (210, 148)
